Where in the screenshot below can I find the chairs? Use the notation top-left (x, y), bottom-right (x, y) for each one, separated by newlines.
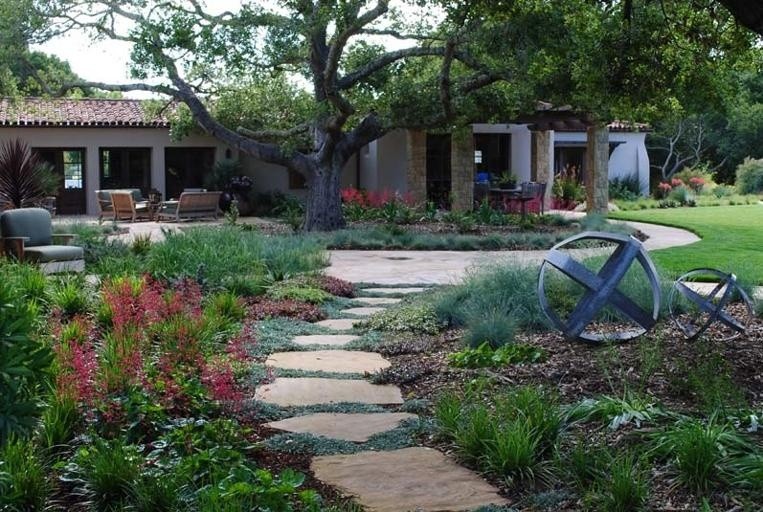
top-left (509, 180), bottom-right (548, 216)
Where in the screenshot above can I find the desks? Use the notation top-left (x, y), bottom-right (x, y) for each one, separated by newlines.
top-left (487, 186), bottom-right (522, 210)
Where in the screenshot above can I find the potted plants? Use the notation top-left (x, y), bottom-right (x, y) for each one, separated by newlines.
top-left (497, 169), bottom-right (522, 189)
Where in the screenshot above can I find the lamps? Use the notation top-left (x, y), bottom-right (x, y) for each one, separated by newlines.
top-left (474, 150), bottom-right (482, 164)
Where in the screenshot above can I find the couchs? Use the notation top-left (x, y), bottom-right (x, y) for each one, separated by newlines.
top-left (107, 191), bottom-right (149, 224)
top-left (155, 192), bottom-right (223, 225)
top-left (0, 207), bottom-right (85, 276)
top-left (183, 186), bottom-right (205, 192)
top-left (93, 188), bottom-right (148, 222)
